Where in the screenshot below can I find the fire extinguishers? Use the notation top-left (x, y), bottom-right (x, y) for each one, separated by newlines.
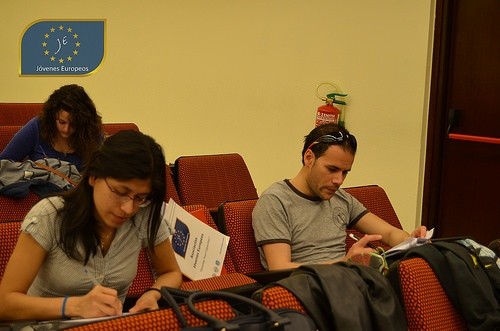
top-left (315, 92), bottom-right (349, 130)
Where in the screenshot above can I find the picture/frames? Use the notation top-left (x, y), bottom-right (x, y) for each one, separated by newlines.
top-left (18, 18), bottom-right (107, 78)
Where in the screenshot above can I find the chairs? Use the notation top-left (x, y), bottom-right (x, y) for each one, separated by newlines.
top-left (262, 287), bottom-right (307, 314)
top-left (0, 220), bottom-right (24, 280)
top-left (124, 246), bottom-right (155, 297)
top-left (174, 152), bottom-right (259, 212)
top-left (61, 299), bottom-right (236, 331)
top-left (0, 191), bottom-right (43, 223)
top-left (101, 121), bottom-right (142, 140)
top-left (0, 126), bottom-right (26, 152)
top-left (219, 196), bottom-right (295, 283)
top-left (0, 103), bottom-right (47, 125)
top-left (178, 204), bottom-right (258, 295)
top-left (343, 184), bottom-right (406, 256)
top-left (398, 257), bottom-right (467, 331)
top-left (165, 163), bottom-right (184, 206)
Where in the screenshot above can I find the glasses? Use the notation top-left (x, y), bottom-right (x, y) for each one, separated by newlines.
top-left (308, 130), bottom-right (355, 148)
top-left (104, 177), bottom-right (152, 208)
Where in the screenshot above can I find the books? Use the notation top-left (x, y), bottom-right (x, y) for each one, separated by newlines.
top-left (154, 198), bottom-right (230, 281)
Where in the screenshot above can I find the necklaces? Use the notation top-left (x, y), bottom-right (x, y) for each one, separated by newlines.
top-left (98, 228), bottom-right (113, 248)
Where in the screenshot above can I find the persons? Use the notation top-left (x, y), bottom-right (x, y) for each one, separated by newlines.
top-left (0, 84), bottom-right (104, 174)
top-left (0, 131), bottom-right (182, 320)
top-left (252, 121), bottom-right (427, 271)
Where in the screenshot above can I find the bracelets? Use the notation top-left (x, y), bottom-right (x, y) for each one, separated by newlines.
top-left (142, 288), bottom-right (161, 295)
top-left (62, 296), bottom-right (71, 320)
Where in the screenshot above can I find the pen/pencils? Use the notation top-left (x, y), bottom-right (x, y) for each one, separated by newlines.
top-left (348, 233), bottom-right (377, 250)
top-left (39, 145), bottom-right (47, 157)
top-left (84, 269), bottom-right (101, 287)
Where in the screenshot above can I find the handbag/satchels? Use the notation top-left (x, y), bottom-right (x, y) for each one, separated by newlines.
top-left (161, 286), bottom-right (319, 331)
